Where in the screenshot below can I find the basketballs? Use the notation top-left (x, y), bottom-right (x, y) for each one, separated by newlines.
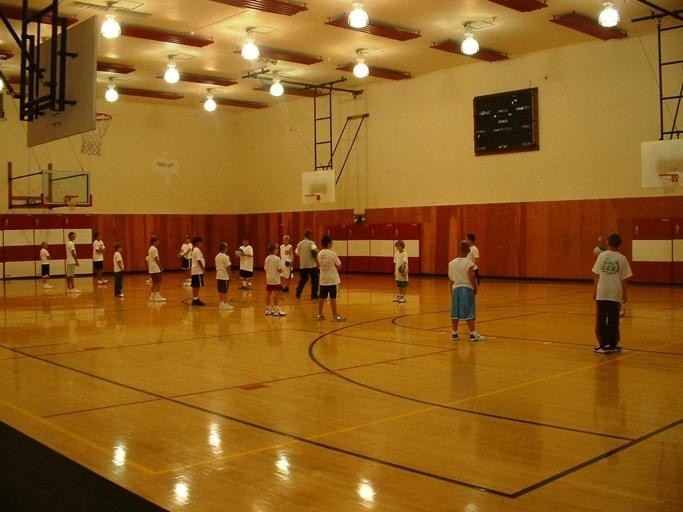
top-left (399, 265), bottom-right (407, 274)
top-left (284, 259), bottom-right (291, 266)
top-left (235, 248), bottom-right (244, 257)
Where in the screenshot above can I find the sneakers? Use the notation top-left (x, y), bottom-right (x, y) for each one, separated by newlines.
top-left (469, 332), bottom-right (484, 341)
top-left (392, 294), bottom-right (406, 303)
top-left (452, 334), bottom-right (460, 340)
top-left (149, 296), bottom-right (166, 301)
top-left (97, 279), bottom-right (108, 284)
top-left (318, 314), bottom-right (346, 322)
top-left (239, 285), bottom-right (253, 290)
top-left (43, 284), bottom-right (81, 293)
top-left (219, 304), bottom-right (234, 310)
top-left (594, 345), bottom-right (623, 353)
top-left (265, 309), bottom-right (286, 316)
top-left (192, 299), bottom-right (204, 305)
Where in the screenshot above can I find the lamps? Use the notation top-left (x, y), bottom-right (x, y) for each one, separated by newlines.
top-left (100, 5), bottom-right (121, 39)
top-left (104, 78), bottom-right (120, 102)
top-left (203, 88), bottom-right (216, 112)
top-left (347, 0), bottom-right (370, 28)
top-left (163, 57), bottom-right (180, 84)
top-left (241, 29), bottom-right (260, 60)
top-left (269, 71), bottom-right (284, 96)
top-left (598, 1), bottom-right (620, 27)
top-left (460, 22), bottom-right (480, 55)
top-left (352, 48), bottom-right (370, 78)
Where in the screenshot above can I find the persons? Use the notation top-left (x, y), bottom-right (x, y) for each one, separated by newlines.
top-left (447, 347), bottom-right (480, 444)
top-left (592, 245), bottom-right (625, 325)
top-left (145, 235), bottom-right (167, 301)
top-left (391, 240), bottom-right (409, 304)
top-left (215, 240), bottom-right (235, 309)
top-left (446, 240), bottom-right (486, 341)
top-left (40, 242), bottom-right (52, 288)
top-left (177, 234), bottom-right (206, 305)
top-left (264, 229), bottom-right (346, 323)
top-left (465, 233), bottom-right (480, 286)
top-left (239, 236), bottom-right (253, 290)
top-left (592, 353), bottom-right (629, 470)
top-left (66, 232), bottom-right (83, 293)
top-left (113, 242), bottom-right (125, 297)
top-left (93, 231), bottom-right (108, 284)
top-left (591, 233), bottom-right (634, 354)
